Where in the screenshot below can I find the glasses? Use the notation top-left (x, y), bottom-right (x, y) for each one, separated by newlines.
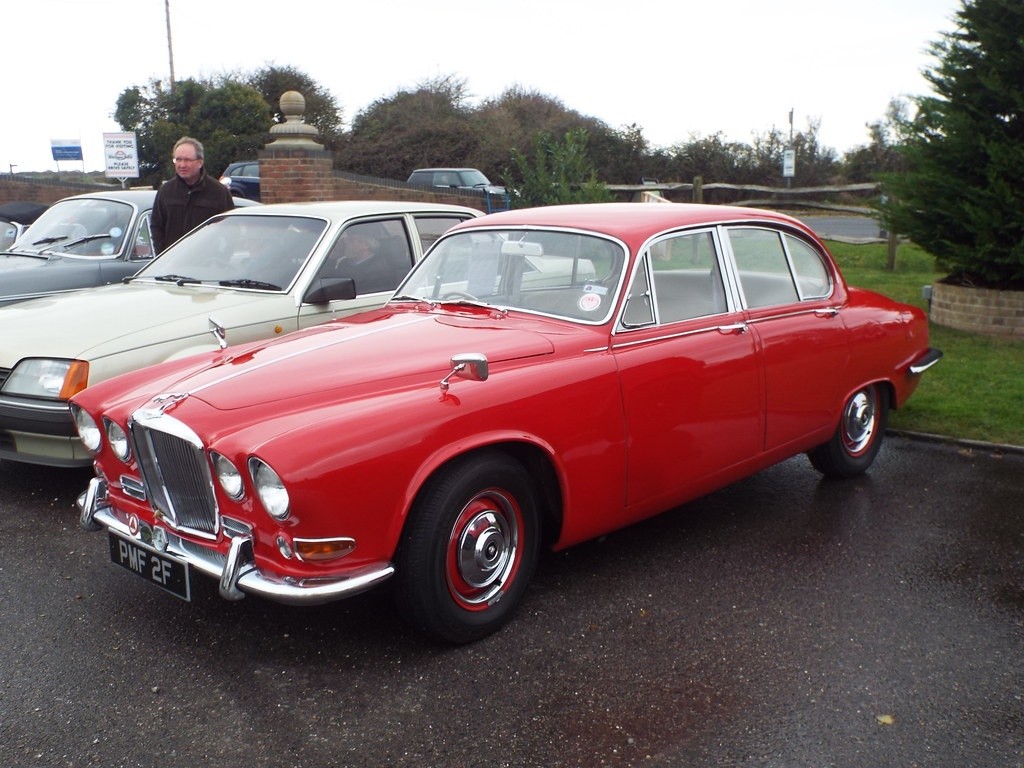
top-left (172, 157), bottom-right (201, 164)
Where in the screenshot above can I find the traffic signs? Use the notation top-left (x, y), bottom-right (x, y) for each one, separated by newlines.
top-left (50, 139), bottom-right (85, 161)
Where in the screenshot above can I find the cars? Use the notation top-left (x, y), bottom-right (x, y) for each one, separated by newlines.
top-left (218, 162), bottom-right (260, 195)
top-left (1, 179), bottom-right (159, 307)
top-left (406, 164), bottom-right (517, 196)
top-left (57, 200), bottom-right (942, 635)
top-left (2, 198), bottom-right (487, 482)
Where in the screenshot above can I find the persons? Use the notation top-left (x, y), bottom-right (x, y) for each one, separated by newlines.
top-left (150, 136), bottom-right (235, 258)
top-left (324, 231), bottom-right (385, 294)
